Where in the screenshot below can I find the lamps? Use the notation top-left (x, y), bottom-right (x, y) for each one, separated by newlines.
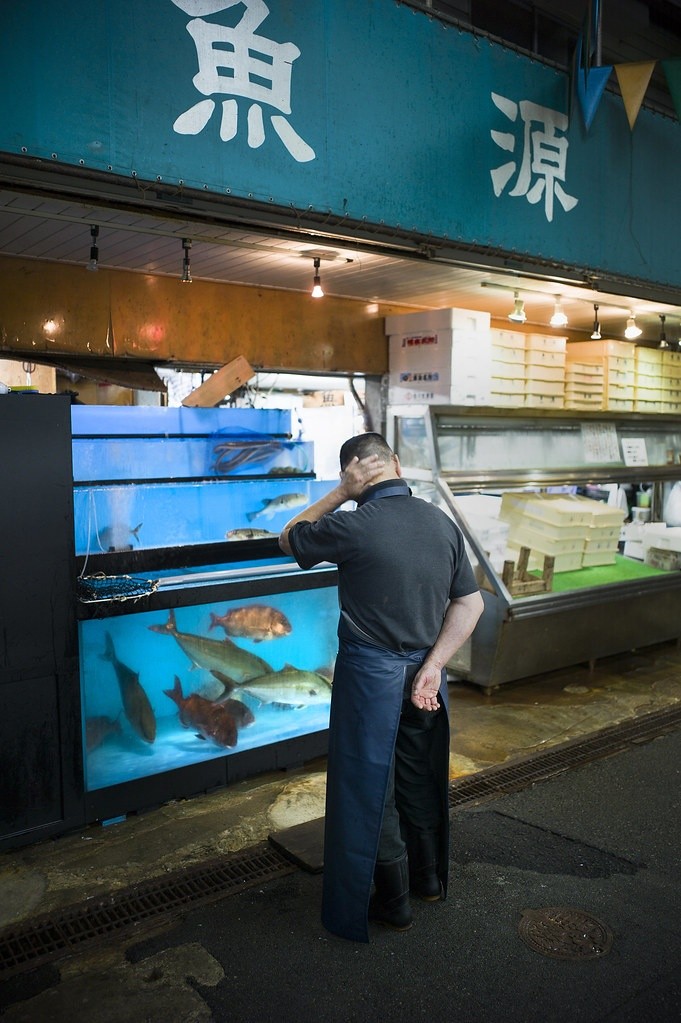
top-left (659, 315), bottom-right (669, 347)
top-left (550, 295), bottom-right (568, 328)
top-left (180, 238), bottom-right (193, 284)
top-left (311, 259), bottom-right (324, 298)
top-left (86, 226), bottom-right (99, 272)
top-left (591, 304), bottom-right (601, 339)
top-left (625, 311), bottom-right (643, 339)
top-left (509, 293), bottom-right (527, 323)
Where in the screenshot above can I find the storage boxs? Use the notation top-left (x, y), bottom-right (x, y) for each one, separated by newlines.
top-left (384, 306), bottom-right (681, 415)
top-left (498, 491), bottom-right (681, 572)
top-left (69, 405), bottom-right (337, 825)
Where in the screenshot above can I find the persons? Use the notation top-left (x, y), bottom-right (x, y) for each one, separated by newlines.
top-left (278, 432), bottom-right (484, 944)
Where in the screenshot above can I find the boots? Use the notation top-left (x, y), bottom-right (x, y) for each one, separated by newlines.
top-left (406, 830), bottom-right (442, 901)
top-left (367, 851), bottom-right (413, 930)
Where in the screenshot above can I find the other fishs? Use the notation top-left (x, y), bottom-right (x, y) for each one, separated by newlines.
top-left (97, 632), bottom-right (157, 744)
top-left (148, 606), bottom-right (274, 682)
top-left (209, 662), bottom-right (332, 710)
top-left (226, 527), bottom-right (278, 540)
top-left (163, 675), bottom-right (255, 746)
top-left (95, 522), bottom-right (143, 545)
top-left (85, 715), bottom-right (124, 754)
top-left (207, 606), bottom-right (292, 642)
top-left (246, 494), bottom-right (308, 524)
top-left (208, 441), bottom-right (281, 475)
